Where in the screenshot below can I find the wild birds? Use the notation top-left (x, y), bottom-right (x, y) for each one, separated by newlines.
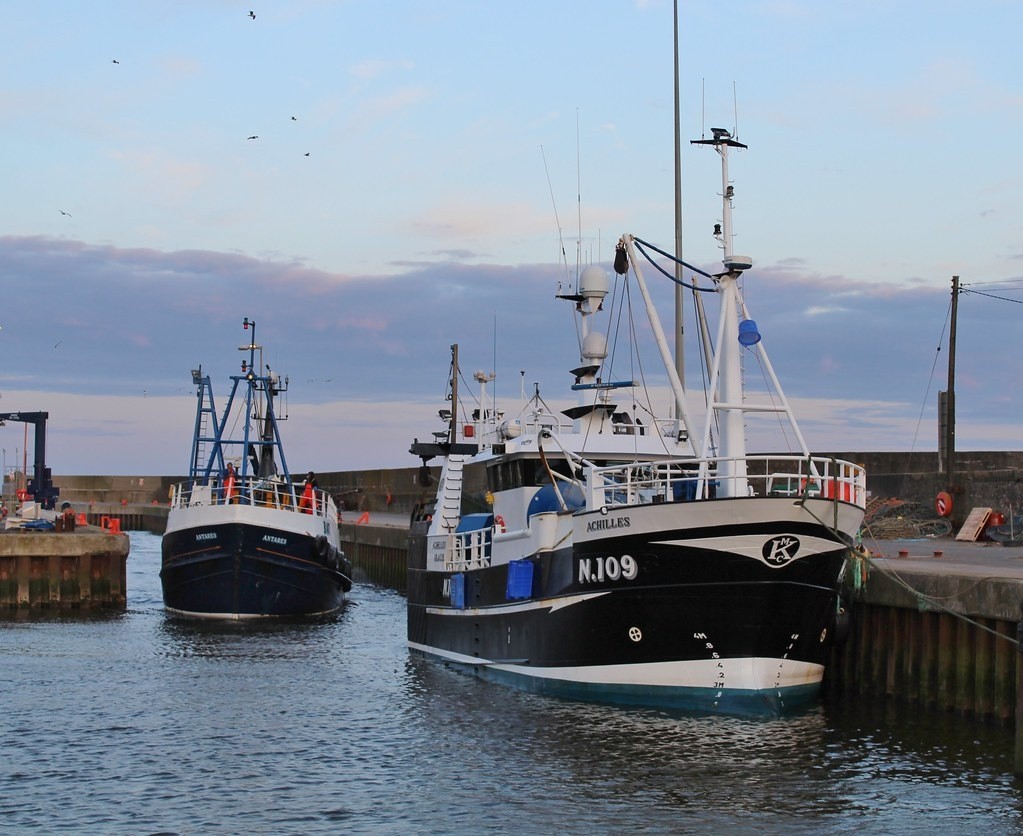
top-left (112, 60), bottom-right (120, 65)
top-left (247, 11), bottom-right (256, 22)
top-left (56, 209), bottom-right (73, 218)
top-left (247, 116), bottom-right (310, 157)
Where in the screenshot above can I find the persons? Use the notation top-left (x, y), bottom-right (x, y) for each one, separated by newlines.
top-left (297, 471), bottom-right (321, 515)
top-left (220, 462), bottom-right (238, 504)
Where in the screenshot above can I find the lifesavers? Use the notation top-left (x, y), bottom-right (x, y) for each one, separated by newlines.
top-left (494, 515), bottom-right (505, 534)
top-left (167, 487), bottom-right (172, 498)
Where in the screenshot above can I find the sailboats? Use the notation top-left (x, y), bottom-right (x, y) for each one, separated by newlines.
top-left (404, 1), bottom-right (867, 716)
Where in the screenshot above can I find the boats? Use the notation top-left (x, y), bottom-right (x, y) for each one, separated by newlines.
top-left (159, 317), bottom-right (354, 620)
